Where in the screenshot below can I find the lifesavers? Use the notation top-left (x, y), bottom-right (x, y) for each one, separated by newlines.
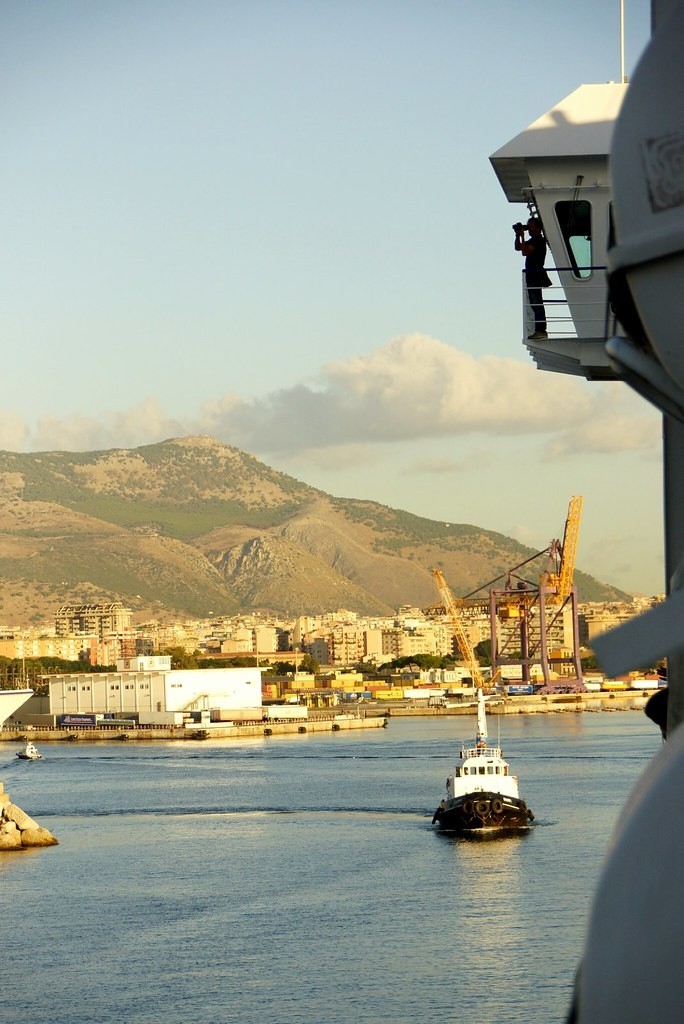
top-left (519, 800), bottom-right (527, 811)
top-left (463, 800), bottom-right (474, 814)
top-left (475, 801), bottom-right (490, 815)
top-left (491, 798), bottom-right (503, 814)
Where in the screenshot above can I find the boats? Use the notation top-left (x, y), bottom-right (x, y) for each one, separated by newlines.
top-left (16, 741), bottom-right (42, 759)
top-left (438, 687), bottom-right (527, 831)
